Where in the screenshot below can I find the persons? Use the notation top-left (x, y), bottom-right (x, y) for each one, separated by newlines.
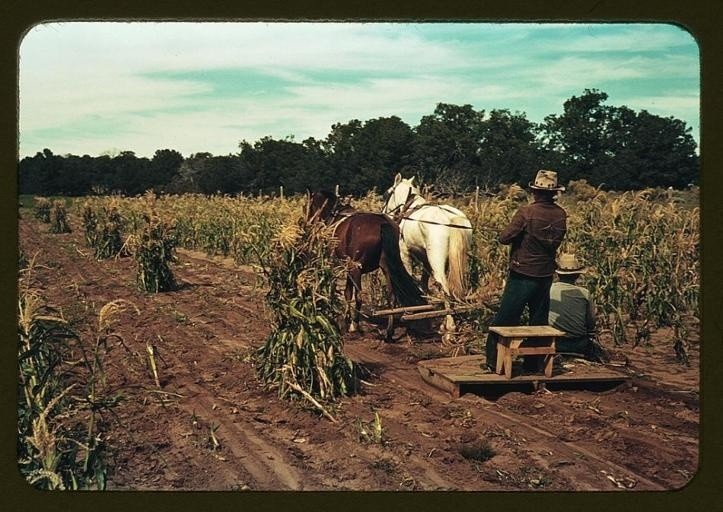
top-left (478, 169), bottom-right (567, 377)
top-left (547, 253), bottom-right (604, 367)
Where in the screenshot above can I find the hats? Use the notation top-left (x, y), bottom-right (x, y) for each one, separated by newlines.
top-left (555, 254), bottom-right (590, 275)
top-left (528, 169), bottom-right (566, 192)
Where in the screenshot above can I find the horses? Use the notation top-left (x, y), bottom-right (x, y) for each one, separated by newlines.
top-left (297, 182), bottom-right (429, 345)
top-left (380, 171), bottom-right (475, 348)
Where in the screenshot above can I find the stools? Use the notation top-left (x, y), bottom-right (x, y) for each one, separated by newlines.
top-left (488, 325), bottom-right (566, 379)
